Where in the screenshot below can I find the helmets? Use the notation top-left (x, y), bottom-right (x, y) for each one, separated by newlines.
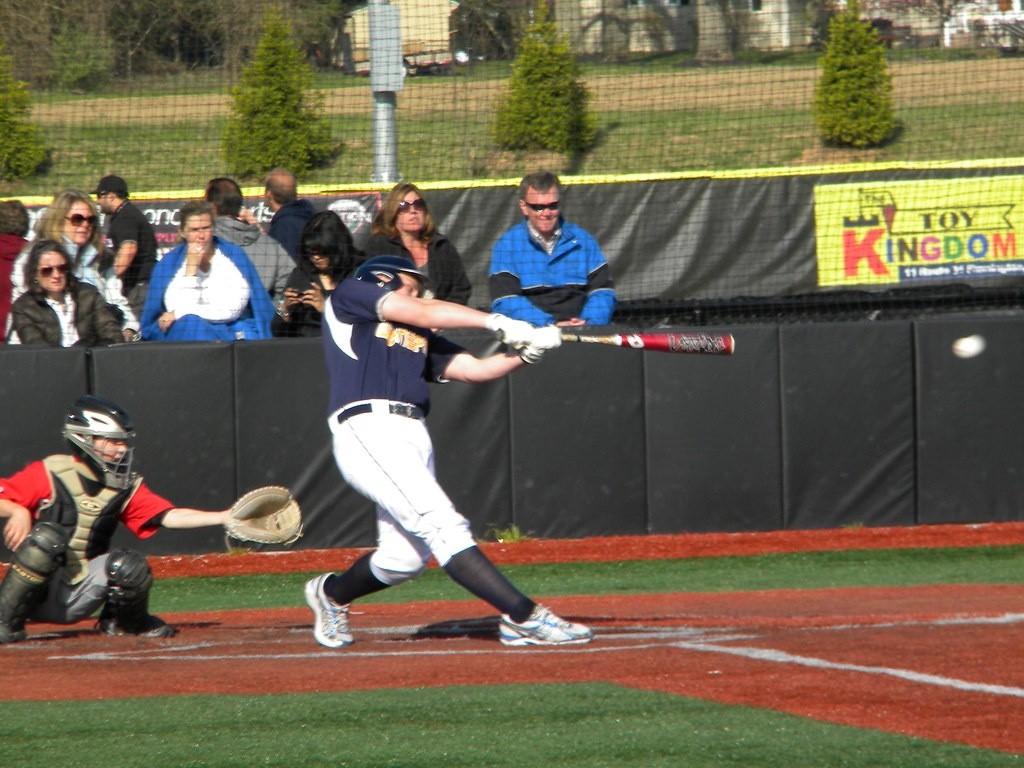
top-left (355, 254), bottom-right (434, 303)
top-left (62, 394), bottom-right (135, 489)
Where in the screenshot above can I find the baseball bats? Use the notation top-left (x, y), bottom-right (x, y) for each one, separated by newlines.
top-left (494, 329), bottom-right (735, 354)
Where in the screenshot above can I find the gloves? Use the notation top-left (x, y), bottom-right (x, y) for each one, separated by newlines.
top-left (519, 323), bottom-right (563, 366)
top-left (485, 311), bottom-right (538, 351)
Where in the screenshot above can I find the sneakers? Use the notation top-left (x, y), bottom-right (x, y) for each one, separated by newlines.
top-left (497, 597), bottom-right (593, 647)
top-left (99, 613), bottom-right (174, 642)
top-left (305, 573), bottom-right (364, 646)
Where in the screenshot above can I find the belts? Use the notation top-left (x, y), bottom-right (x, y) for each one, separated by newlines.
top-left (338, 404), bottom-right (424, 425)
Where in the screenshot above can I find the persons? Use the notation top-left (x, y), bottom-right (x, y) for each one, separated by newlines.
top-left (488, 172), bottom-right (617, 328)
top-left (365, 183), bottom-right (472, 306)
top-left (271, 210), bottom-right (365, 337)
top-left (239, 171), bottom-right (316, 262)
top-left (13, 240), bottom-right (124, 346)
top-left (140, 200), bottom-right (275, 340)
top-left (0, 394), bottom-right (304, 643)
top-left (304, 255), bottom-right (595, 649)
top-left (205, 177), bottom-right (297, 306)
top-left (4, 190), bottom-right (140, 345)
top-left (0, 199), bottom-right (29, 345)
top-left (90, 176), bottom-right (158, 321)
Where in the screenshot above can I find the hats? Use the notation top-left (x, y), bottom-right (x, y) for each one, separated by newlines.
top-left (89, 175), bottom-right (127, 197)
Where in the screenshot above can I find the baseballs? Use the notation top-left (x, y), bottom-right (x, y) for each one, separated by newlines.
top-left (952, 334), bottom-right (987, 358)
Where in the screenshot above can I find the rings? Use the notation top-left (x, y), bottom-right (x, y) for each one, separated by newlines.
top-left (196, 247), bottom-right (201, 251)
top-left (163, 320), bottom-right (167, 322)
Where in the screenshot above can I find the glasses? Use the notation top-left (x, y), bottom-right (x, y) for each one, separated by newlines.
top-left (35, 264), bottom-right (70, 278)
top-left (398, 199), bottom-right (426, 214)
top-left (525, 199), bottom-right (559, 213)
top-left (65, 214), bottom-right (97, 227)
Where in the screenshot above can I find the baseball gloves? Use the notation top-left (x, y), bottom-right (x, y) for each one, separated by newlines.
top-left (222, 486), bottom-right (303, 545)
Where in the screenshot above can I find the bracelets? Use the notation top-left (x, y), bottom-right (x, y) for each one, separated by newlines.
top-left (276, 307), bottom-right (289, 317)
